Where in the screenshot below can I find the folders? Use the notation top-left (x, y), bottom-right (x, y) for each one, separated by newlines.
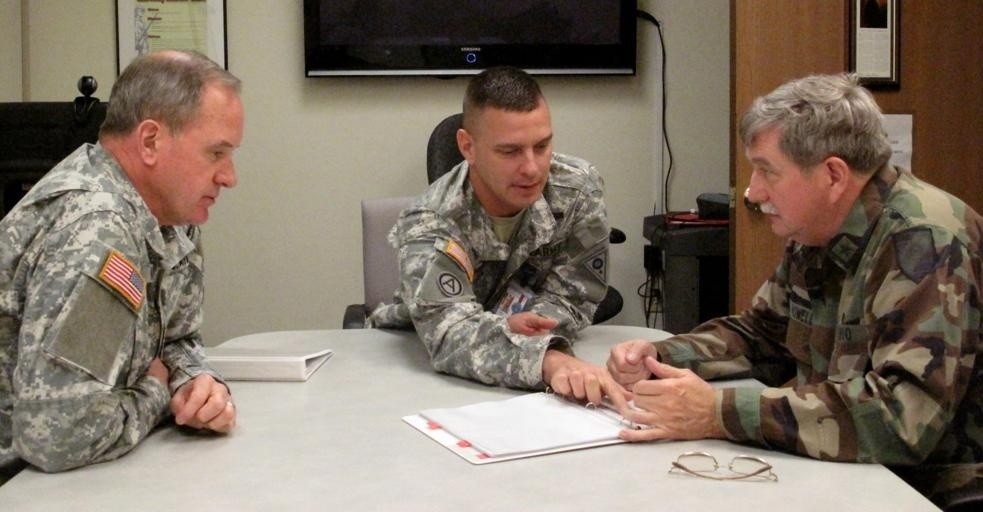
top-left (204, 348), bottom-right (335, 381)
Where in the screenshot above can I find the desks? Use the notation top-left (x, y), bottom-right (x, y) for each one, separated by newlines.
top-left (0, 327), bottom-right (943, 511)
top-left (643, 210), bottom-right (729, 335)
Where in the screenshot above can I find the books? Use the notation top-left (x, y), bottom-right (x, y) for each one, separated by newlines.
top-left (402, 391), bottom-right (652, 464)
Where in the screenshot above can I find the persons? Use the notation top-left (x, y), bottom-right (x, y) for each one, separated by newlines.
top-left (364, 70), bottom-right (634, 412)
top-left (0, 51), bottom-right (244, 474)
top-left (607, 70), bottom-right (983, 512)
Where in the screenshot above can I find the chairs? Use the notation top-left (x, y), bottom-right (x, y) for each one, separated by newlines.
top-left (427, 114), bottom-right (626, 327)
top-left (343, 198), bottom-right (421, 329)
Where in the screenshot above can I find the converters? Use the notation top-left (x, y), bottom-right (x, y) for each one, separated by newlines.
top-left (643, 244), bottom-right (662, 271)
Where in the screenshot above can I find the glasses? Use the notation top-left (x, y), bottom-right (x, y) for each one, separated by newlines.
top-left (668, 449), bottom-right (780, 487)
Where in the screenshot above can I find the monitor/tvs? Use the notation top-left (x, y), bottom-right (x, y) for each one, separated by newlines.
top-left (303, 0), bottom-right (637, 76)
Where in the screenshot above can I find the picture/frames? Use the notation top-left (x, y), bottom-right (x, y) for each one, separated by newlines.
top-left (849, 0), bottom-right (901, 92)
top-left (115, 0), bottom-right (228, 75)
